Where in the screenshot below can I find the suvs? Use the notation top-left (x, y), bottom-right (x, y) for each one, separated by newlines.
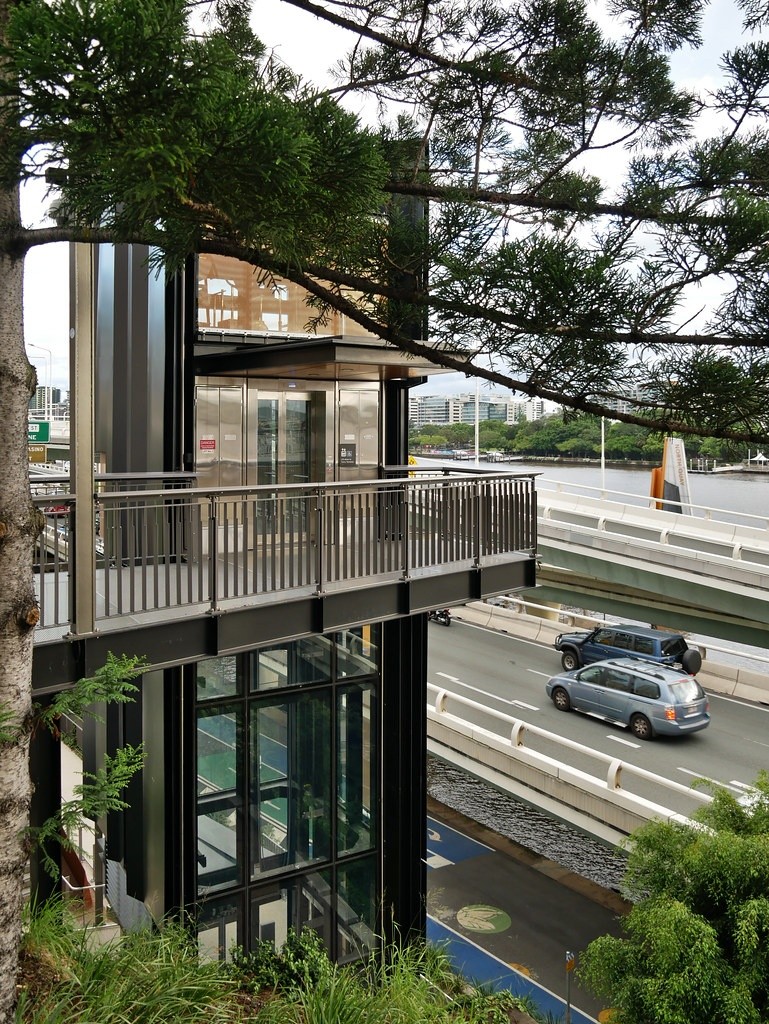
top-left (546, 655), bottom-right (711, 741)
top-left (552, 625), bottom-right (702, 680)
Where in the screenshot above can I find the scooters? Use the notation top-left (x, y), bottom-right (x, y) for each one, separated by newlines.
top-left (425, 609), bottom-right (453, 625)
